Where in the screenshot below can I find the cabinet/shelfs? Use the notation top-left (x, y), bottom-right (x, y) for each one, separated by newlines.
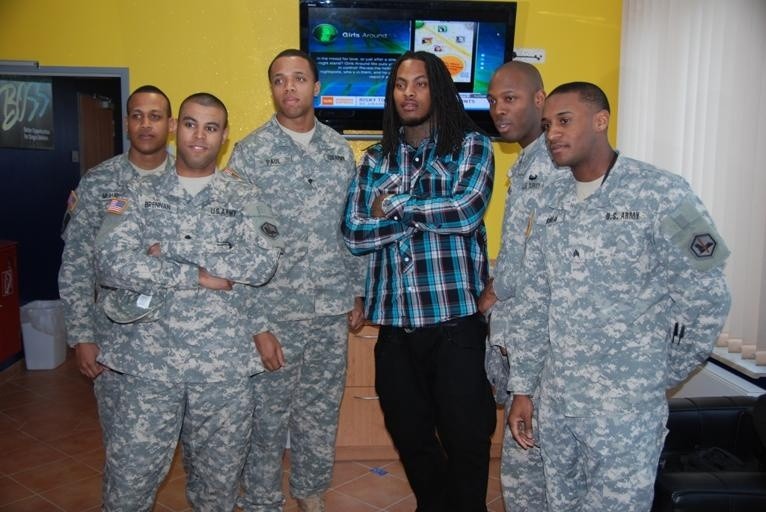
top-left (671, 345), bottom-right (766, 399)
top-left (334, 315), bottom-right (505, 461)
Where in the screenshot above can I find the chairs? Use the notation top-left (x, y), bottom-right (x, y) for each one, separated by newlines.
top-left (651, 394), bottom-right (766, 512)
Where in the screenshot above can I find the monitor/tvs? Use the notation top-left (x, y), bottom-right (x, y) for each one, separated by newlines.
top-left (299, 1), bottom-right (517, 134)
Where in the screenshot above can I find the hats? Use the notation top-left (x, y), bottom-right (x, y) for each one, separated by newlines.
top-left (102, 287), bottom-right (169, 325)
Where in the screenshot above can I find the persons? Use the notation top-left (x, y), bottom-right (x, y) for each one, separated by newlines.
top-left (479, 62), bottom-right (558, 512)
top-left (225, 47), bottom-right (371, 512)
top-left (501, 79), bottom-right (733, 512)
top-left (341, 49), bottom-right (497, 512)
top-left (56, 85), bottom-right (177, 449)
top-left (92, 92), bottom-right (281, 512)
